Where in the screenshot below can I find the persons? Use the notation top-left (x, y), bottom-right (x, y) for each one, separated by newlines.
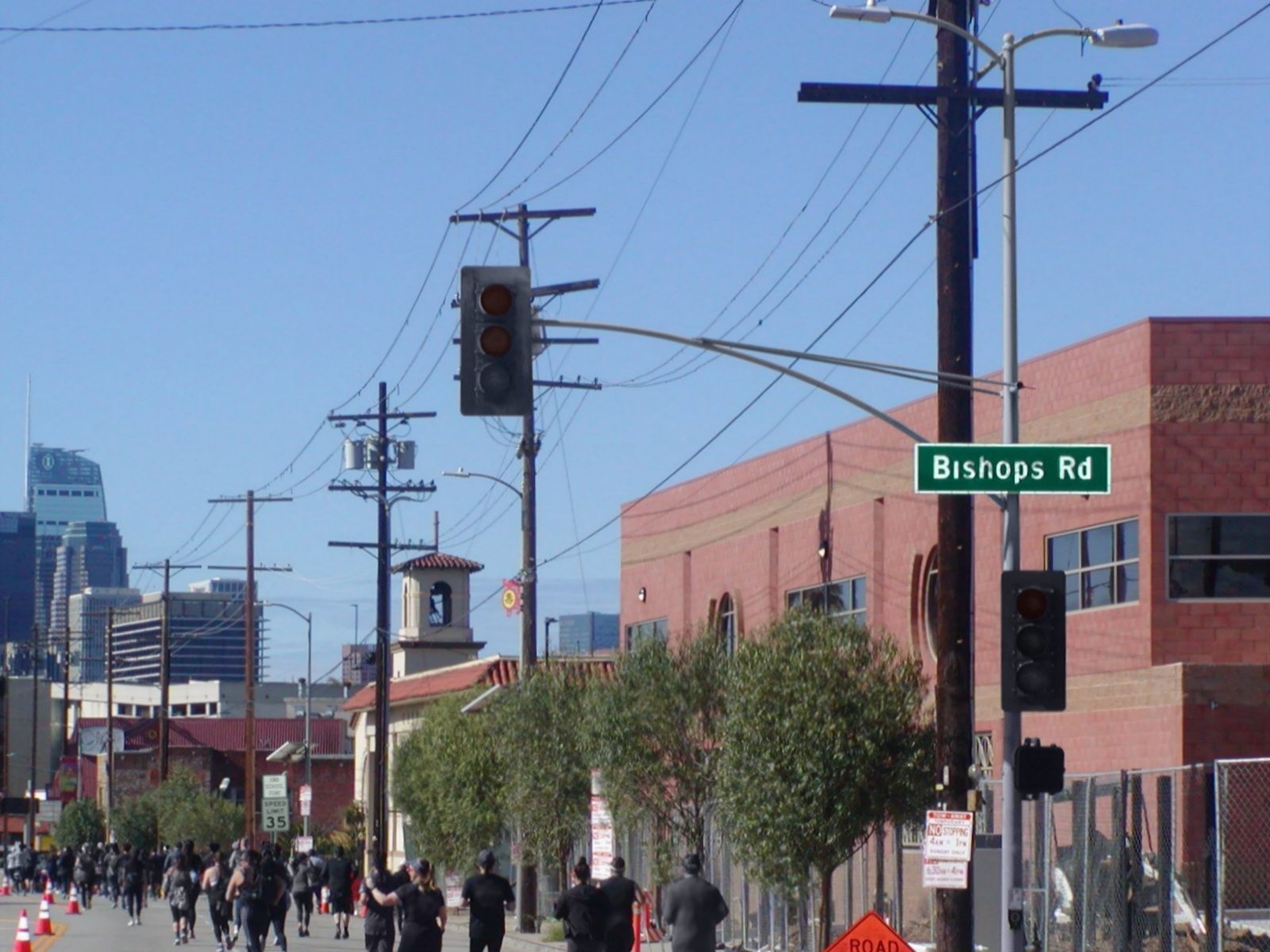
top-left (659, 853), bottom-right (729, 952)
top-left (553, 856), bottom-right (646, 952)
top-left (0, 836), bottom-right (448, 952)
top-left (461, 849), bottom-right (516, 952)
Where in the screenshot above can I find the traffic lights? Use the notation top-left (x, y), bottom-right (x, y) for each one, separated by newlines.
top-left (1013, 743), bottom-right (1064, 795)
top-left (998, 568), bottom-right (1066, 713)
top-left (458, 265), bottom-right (534, 418)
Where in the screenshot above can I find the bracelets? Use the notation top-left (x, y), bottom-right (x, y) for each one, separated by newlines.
top-left (370, 884), bottom-right (376, 890)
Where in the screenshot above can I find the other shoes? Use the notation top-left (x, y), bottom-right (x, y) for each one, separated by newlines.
top-left (335, 930), bottom-right (341, 939)
top-left (180, 933), bottom-right (188, 944)
top-left (190, 930), bottom-right (195, 938)
top-left (298, 927), bottom-right (304, 937)
top-left (216, 943), bottom-right (224, 952)
top-left (226, 938), bottom-right (232, 950)
top-left (304, 931), bottom-right (310, 936)
top-left (112, 902), bottom-right (148, 926)
top-left (173, 938), bottom-right (180, 946)
top-left (343, 927), bottom-right (349, 938)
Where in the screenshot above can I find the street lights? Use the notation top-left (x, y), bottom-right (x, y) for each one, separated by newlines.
top-left (251, 599), bottom-right (313, 860)
top-left (441, 467), bottom-right (528, 682)
top-left (827, 0), bottom-right (1164, 951)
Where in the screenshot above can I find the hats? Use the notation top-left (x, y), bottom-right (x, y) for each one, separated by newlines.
top-left (682, 854), bottom-right (701, 874)
top-left (410, 857), bottom-right (430, 878)
top-left (609, 856), bottom-right (626, 872)
top-left (476, 850), bottom-right (496, 869)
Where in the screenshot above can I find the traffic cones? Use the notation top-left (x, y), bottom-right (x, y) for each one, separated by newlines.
top-left (42, 877), bottom-right (56, 905)
top-left (31, 895), bottom-right (56, 936)
top-left (0, 875), bottom-right (11, 896)
top-left (10, 910), bottom-right (32, 952)
top-left (66, 883), bottom-right (81, 915)
